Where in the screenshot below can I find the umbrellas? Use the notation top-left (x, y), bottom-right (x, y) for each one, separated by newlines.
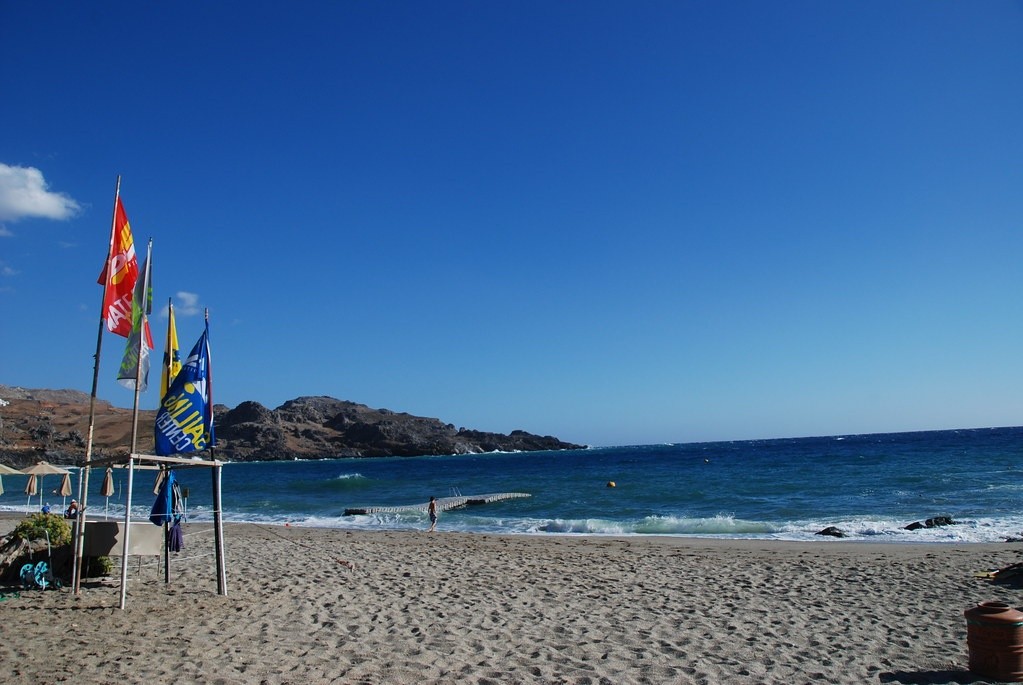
top-left (60, 469), bottom-right (72, 518)
top-left (24, 474), bottom-right (37, 515)
top-left (0, 475), bottom-right (4, 495)
top-left (0, 463), bottom-right (26, 475)
top-left (153, 463), bottom-right (170, 495)
top-left (19, 461), bottom-right (75, 512)
top-left (99, 467), bottom-right (115, 521)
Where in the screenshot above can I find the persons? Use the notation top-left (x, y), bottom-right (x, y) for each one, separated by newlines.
top-left (40, 502), bottom-right (51, 515)
top-left (428, 496), bottom-right (438, 532)
top-left (65, 498), bottom-right (83, 519)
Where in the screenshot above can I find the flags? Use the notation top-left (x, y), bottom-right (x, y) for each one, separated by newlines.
top-left (149, 469), bottom-right (176, 527)
top-left (96, 174), bottom-right (157, 350)
top-left (154, 317), bottom-right (216, 457)
top-left (152, 304), bottom-right (183, 448)
top-left (116, 240), bottom-right (153, 394)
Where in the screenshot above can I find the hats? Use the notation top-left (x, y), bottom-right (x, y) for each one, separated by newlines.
top-left (71, 499), bottom-right (76, 503)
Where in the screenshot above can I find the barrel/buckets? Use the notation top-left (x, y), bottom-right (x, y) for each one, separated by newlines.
top-left (965, 600), bottom-right (1023, 681)
